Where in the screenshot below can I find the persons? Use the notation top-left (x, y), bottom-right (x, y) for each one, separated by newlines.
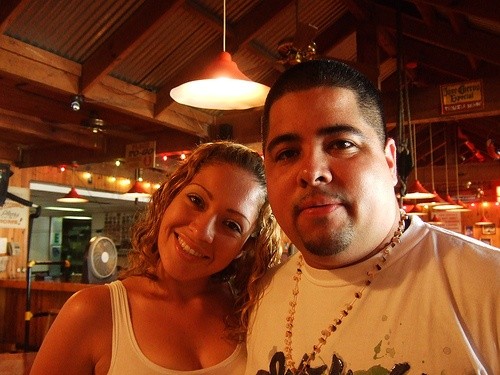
top-left (245, 58), bottom-right (500, 375)
top-left (29, 141), bottom-right (283, 375)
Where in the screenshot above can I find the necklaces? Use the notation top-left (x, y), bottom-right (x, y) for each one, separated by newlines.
top-left (284, 209), bottom-right (409, 375)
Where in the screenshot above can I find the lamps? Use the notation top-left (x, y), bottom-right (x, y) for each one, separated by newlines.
top-left (123, 166), bottom-right (152, 197)
top-left (396, 70), bottom-right (494, 225)
top-left (56, 167), bottom-right (89, 203)
top-left (168, 0), bottom-right (271, 112)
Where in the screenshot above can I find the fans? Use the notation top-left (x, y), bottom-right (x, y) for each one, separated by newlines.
top-left (40, 112), bottom-right (141, 137)
top-left (87, 235), bottom-right (118, 285)
top-left (250, 0), bottom-right (320, 70)
